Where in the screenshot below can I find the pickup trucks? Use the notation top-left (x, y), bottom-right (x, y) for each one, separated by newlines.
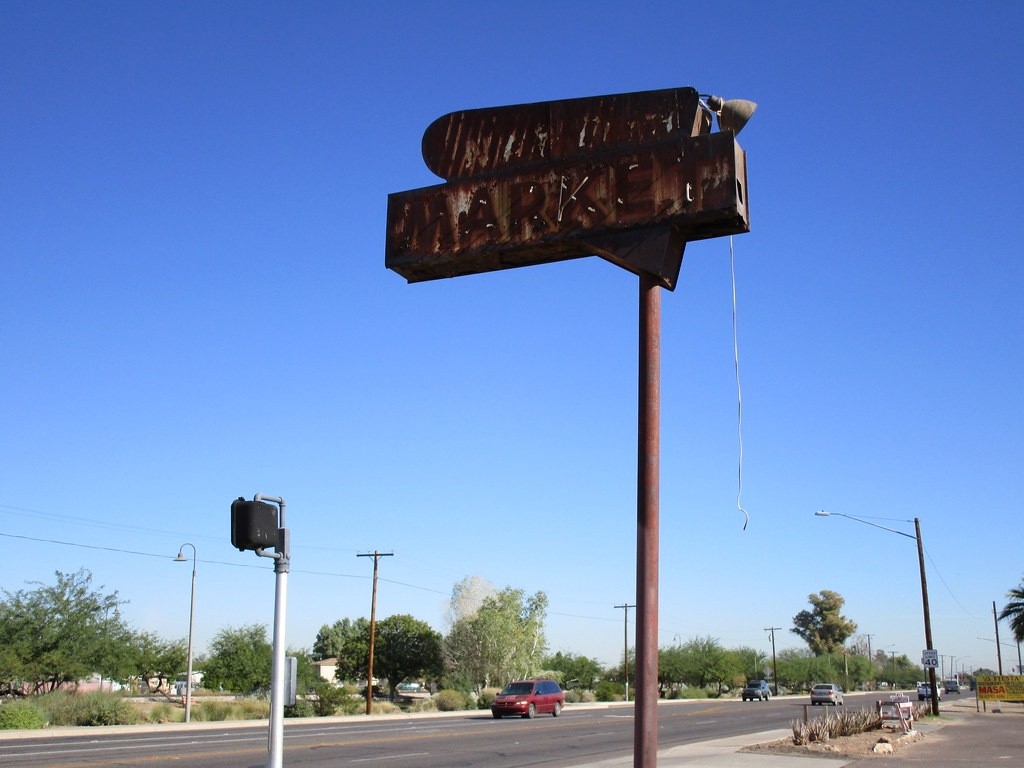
top-left (917, 681), bottom-right (941, 700)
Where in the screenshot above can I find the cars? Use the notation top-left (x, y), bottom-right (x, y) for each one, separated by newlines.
top-left (490, 678), bottom-right (565, 719)
top-left (811, 683), bottom-right (844, 706)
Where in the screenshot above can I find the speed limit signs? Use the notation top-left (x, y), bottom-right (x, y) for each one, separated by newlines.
top-left (922, 649), bottom-right (938, 668)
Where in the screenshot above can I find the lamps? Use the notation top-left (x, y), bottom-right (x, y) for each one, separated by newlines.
top-left (699, 91), bottom-right (758, 137)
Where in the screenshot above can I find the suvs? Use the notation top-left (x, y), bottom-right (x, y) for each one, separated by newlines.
top-left (741, 680), bottom-right (770, 702)
top-left (945, 678), bottom-right (961, 694)
top-left (970, 678), bottom-right (977, 692)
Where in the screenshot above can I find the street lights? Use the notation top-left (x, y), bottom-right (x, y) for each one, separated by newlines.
top-left (172, 542), bottom-right (198, 723)
top-left (977, 636), bottom-right (1022, 676)
top-left (955, 655), bottom-right (972, 672)
top-left (813, 509), bottom-right (939, 717)
